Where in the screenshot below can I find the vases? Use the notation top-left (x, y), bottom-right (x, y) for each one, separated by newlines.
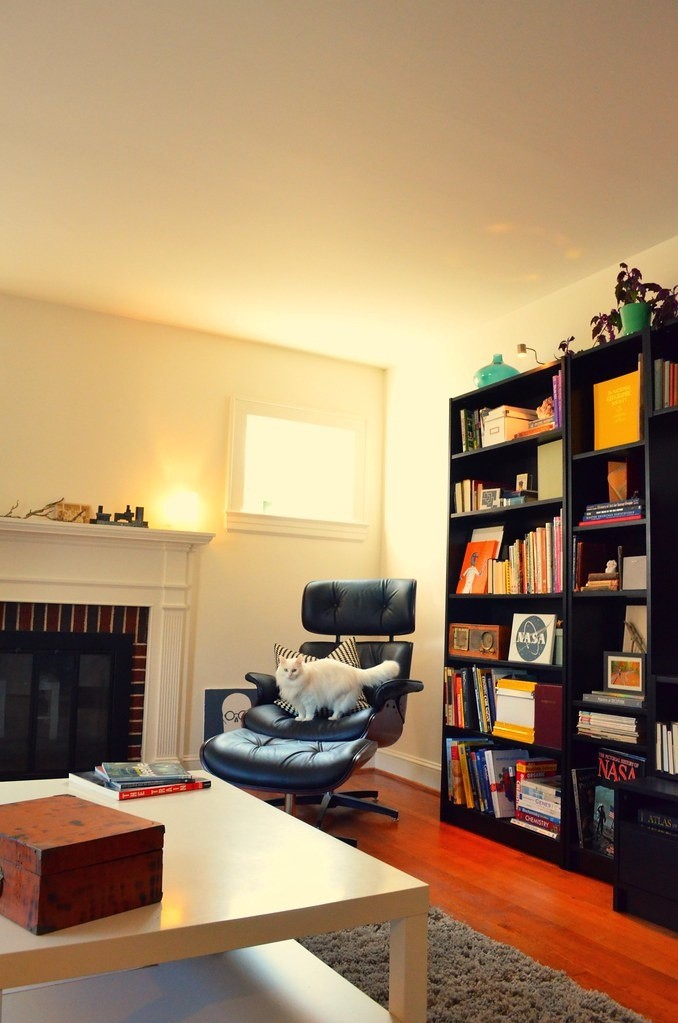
top-left (473, 353), bottom-right (520, 388)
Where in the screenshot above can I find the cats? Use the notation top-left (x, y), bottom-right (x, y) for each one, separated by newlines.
top-left (275, 655), bottom-right (401, 721)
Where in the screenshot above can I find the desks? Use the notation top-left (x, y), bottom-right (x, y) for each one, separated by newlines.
top-left (0, 769), bottom-right (431, 1023)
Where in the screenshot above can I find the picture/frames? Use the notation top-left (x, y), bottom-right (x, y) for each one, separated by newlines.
top-left (602, 651), bottom-right (645, 695)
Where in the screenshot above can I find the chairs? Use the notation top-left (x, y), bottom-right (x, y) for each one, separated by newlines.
top-left (200, 577), bottom-right (424, 847)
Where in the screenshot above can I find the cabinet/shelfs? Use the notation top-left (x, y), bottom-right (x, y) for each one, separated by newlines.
top-left (439, 318), bottom-right (678, 886)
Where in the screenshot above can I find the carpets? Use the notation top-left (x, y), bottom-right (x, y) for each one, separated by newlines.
top-left (295, 907), bottom-right (651, 1023)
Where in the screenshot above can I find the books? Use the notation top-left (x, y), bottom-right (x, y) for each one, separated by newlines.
top-left (513, 369), bottom-right (563, 440)
top-left (444, 665), bottom-right (527, 734)
top-left (652, 357), bottom-right (678, 410)
top-left (582, 691), bottom-right (645, 709)
top-left (69, 761), bottom-right (212, 801)
top-left (572, 535), bottom-right (597, 591)
top-left (576, 711), bottom-right (640, 743)
top-left (459, 406), bottom-right (493, 453)
top-left (571, 747), bottom-right (647, 861)
top-left (634, 804), bottom-right (678, 839)
top-left (577, 499), bottom-right (643, 526)
top-left (446, 737), bottom-right (561, 841)
top-left (654, 720), bottom-right (678, 775)
top-left (455, 480), bottom-right (503, 513)
top-left (456, 509), bottom-right (562, 594)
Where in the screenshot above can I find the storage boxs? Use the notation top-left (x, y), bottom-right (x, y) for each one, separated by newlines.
top-left (0, 794), bottom-right (164, 936)
top-left (537, 439), bottom-right (562, 500)
top-left (613, 776), bottom-right (678, 935)
top-left (534, 684), bottom-right (563, 748)
top-left (553, 629), bottom-right (563, 665)
top-left (481, 405), bottom-right (537, 448)
top-left (492, 679), bottom-right (538, 745)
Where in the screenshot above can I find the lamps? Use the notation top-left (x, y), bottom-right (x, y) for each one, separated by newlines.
top-left (517, 344), bottom-right (544, 365)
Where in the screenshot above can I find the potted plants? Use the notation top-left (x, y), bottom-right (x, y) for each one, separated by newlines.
top-left (554, 260), bottom-right (678, 361)
